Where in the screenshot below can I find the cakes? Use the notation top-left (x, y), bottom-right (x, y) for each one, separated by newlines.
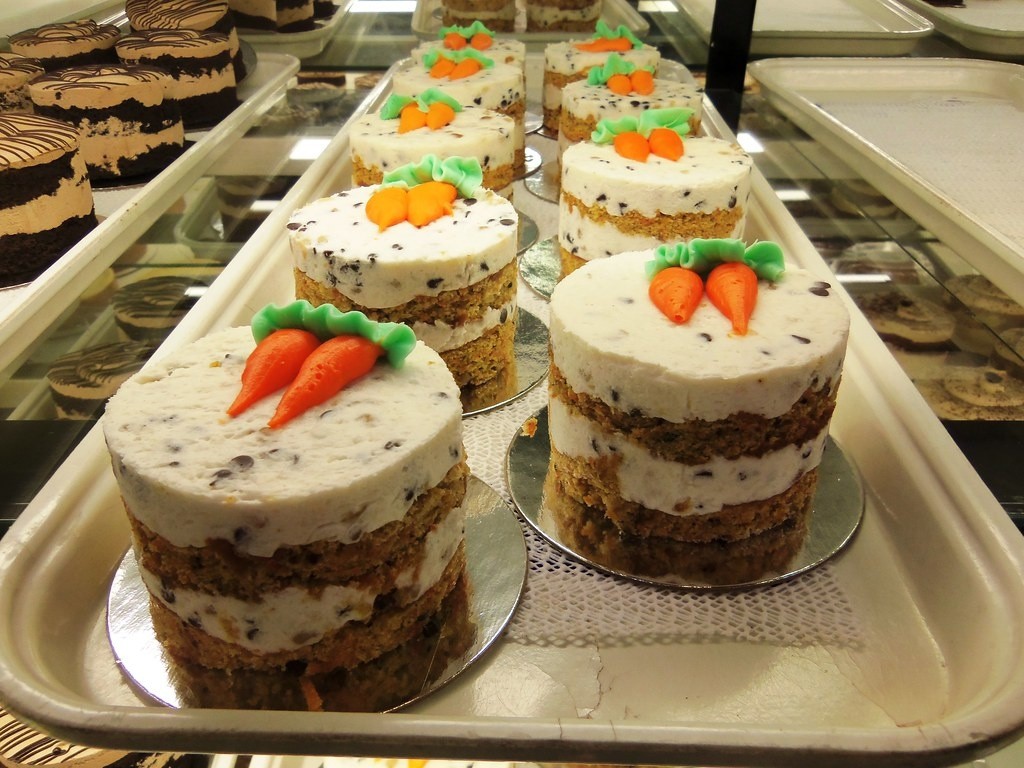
top-left (0, 0), bottom-right (332, 293)
top-left (44, 265), bottom-right (211, 420)
top-left (103, 0), bottom-right (852, 715)
